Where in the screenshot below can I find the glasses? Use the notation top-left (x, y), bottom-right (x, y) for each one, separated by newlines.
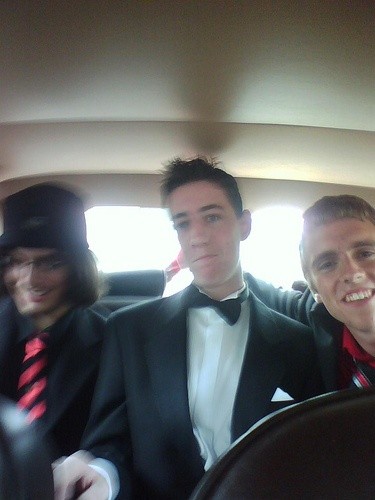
top-left (0, 254), bottom-right (73, 270)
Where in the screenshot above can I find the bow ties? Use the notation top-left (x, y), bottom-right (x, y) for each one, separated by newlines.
top-left (181, 280), bottom-right (249, 326)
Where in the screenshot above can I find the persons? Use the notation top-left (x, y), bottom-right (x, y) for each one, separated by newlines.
top-left (0, 183), bottom-right (106, 463)
top-left (241, 196), bottom-right (375, 391)
top-left (53, 159), bottom-right (324, 499)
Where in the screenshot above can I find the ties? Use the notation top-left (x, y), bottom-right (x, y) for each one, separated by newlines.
top-left (17, 332), bottom-right (49, 426)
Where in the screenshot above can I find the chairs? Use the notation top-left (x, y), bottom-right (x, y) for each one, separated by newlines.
top-left (191, 383), bottom-right (375, 500)
top-left (97, 269), bottom-right (167, 312)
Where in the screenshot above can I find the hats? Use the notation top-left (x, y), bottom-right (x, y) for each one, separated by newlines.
top-left (0, 184), bottom-right (89, 251)
